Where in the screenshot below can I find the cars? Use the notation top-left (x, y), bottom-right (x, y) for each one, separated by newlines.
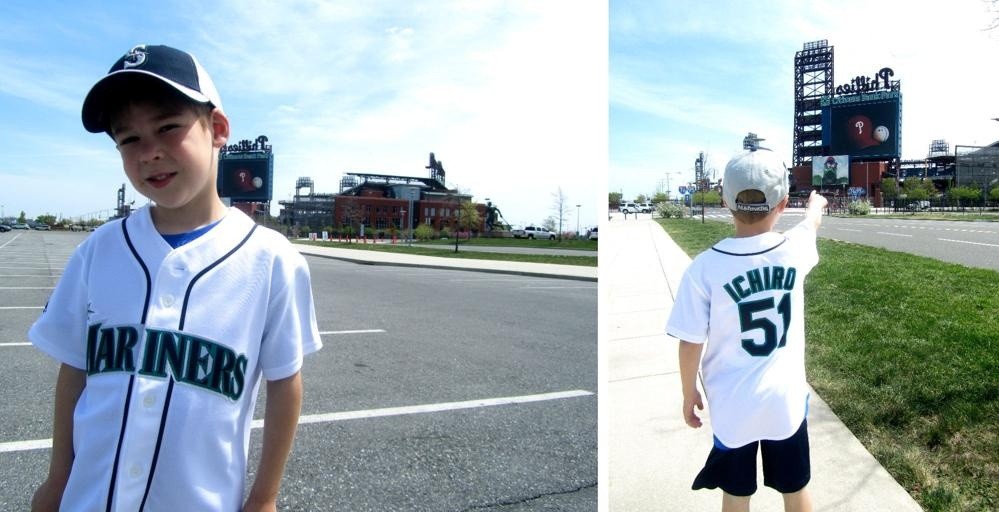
top-left (589, 227), bottom-right (598, 240)
top-left (0, 223), bottom-right (50, 232)
top-left (619, 202), bottom-right (655, 214)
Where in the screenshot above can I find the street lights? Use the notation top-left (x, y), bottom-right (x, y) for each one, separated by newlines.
top-left (576, 205), bottom-right (581, 236)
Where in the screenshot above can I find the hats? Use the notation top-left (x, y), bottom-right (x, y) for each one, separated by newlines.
top-left (844, 114), bottom-right (882, 151)
top-left (720, 144), bottom-right (791, 215)
top-left (79, 43), bottom-right (223, 136)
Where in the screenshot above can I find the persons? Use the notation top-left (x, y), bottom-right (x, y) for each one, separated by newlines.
top-left (25, 43), bottom-right (323, 511)
top-left (664, 147), bottom-right (828, 512)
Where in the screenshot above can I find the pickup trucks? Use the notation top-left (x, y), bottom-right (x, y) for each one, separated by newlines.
top-left (512, 226), bottom-right (556, 240)
